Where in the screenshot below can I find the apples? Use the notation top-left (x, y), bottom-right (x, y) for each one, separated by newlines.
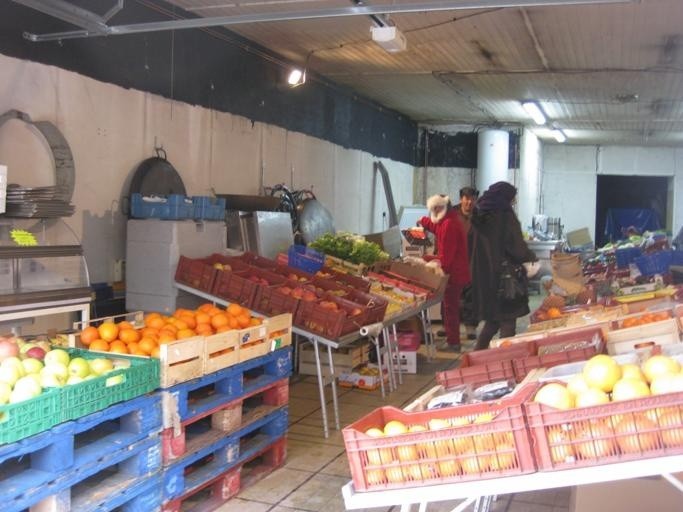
top-left (0, 335), bottom-right (131, 420)
top-left (193, 263), bottom-right (361, 335)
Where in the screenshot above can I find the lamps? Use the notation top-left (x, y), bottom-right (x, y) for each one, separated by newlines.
top-left (522, 99), bottom-right (547, 126)
top-left (287, 54), bottom-right (315, 87)
top-left (552, 129), bottom-right (566, 145)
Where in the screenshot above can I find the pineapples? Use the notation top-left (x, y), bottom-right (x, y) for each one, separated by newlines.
top-left (543, 296), bottom-right (565, 308)
top-left (576, 290), bottom-right (596, 304)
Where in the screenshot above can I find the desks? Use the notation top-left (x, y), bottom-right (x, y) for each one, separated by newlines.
top-left (0, 301), bottom-right (93, 344)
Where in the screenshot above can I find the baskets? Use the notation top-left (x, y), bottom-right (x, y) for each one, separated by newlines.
top-left (176, 235), bottom-right (436, 340)
top-left (343, 297), bottom-right (682, 493)
top-left (1, 345), bottom-right (161, 447)
top-left (0, 192), bottom-right (682, 511)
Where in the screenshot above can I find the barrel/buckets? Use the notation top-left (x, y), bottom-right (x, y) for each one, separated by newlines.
top-left (382, 226), bottom-right (403, 260)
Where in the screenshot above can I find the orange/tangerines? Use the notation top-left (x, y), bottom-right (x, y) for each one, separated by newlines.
top-left (363, 355), bottom-right (683, 484)
top-left (621, 313), bottom-right (671, 327)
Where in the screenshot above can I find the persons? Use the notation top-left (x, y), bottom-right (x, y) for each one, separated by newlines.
top-left (433, 185), bottom-right (480, 340)
top-left (466, 180), bottom-right (542, 352)
top-left (415, 193), bottom-right (471, 353)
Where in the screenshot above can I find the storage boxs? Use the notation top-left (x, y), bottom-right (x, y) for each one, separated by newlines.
top-left (392, 257), bottom-right (450, 291)
top-left (342, 380), bottom-right (542, 493)
top-left (403, 293), bottom-right (682, 413)
top-left (177, 253), bottom-right (436, 342)
top-left (70, 311), bottom-right (294, 389)
top-left (364, 224), bottom-right (402, 260)
top-left (524, 349), bottom-right (682, 473)
